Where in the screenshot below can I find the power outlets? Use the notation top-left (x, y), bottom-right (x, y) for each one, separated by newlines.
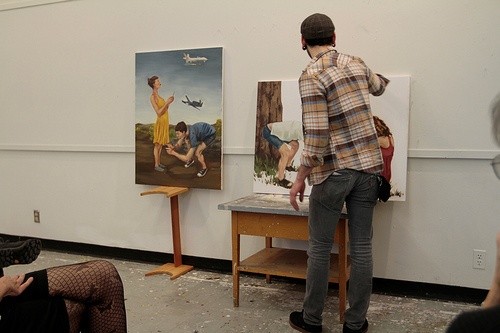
top-left (472, 249), bottom-right (487, 270)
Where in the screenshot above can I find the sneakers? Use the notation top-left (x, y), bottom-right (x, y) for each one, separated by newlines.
top-left (289, 310), bottom-right (322, 333)
top-left (342, 317), bottom-right (368, 333)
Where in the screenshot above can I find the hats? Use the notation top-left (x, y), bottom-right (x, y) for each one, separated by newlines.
top-left (301, 12), bottom-right (335, 39)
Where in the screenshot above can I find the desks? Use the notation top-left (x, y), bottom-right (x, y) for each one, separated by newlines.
top-left (140, 186), bottom-right (193, 280)
top-left (217, 193), bottom-right (351, 324)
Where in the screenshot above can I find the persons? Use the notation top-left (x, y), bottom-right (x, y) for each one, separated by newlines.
top-left (0, 259), bottom-right (127, 333)
top-left (287, 13), bottom-right (390, 332)
top-left (0, 234), bottom-right (42, 277)
top-left (438, 91), bottom-right (500, 333)
